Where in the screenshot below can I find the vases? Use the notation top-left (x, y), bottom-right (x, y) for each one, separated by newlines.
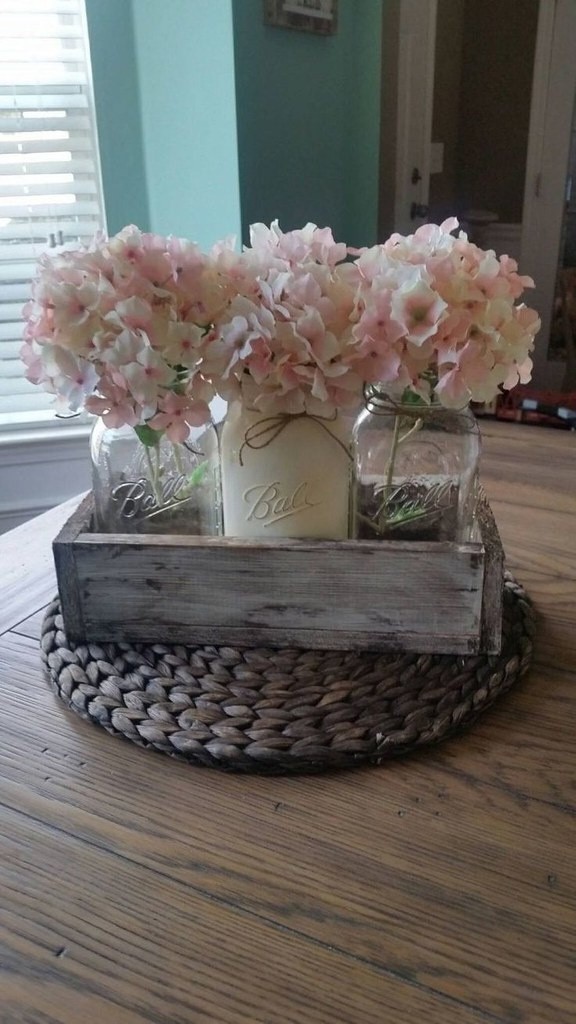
top-left (89, 398), bottom-right (221, 541)
top-left (351, 380), bottom-right (480, 539)
top-left (217, 394), bottom-right (354, 543)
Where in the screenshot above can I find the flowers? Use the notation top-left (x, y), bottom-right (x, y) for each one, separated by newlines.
top-left (21, 222), bottom-right (216, 443)
top-left (352, 218), bottom-right (542, 406)
top-left (206, 220), bottom-right (365, 405)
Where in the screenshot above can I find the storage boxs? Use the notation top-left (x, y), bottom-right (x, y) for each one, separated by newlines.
top-left (54, 487), bottom-right (505, 660)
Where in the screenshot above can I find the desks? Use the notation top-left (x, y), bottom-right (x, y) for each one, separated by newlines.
top-left (0, 411), bottom-right (576, 1024)
